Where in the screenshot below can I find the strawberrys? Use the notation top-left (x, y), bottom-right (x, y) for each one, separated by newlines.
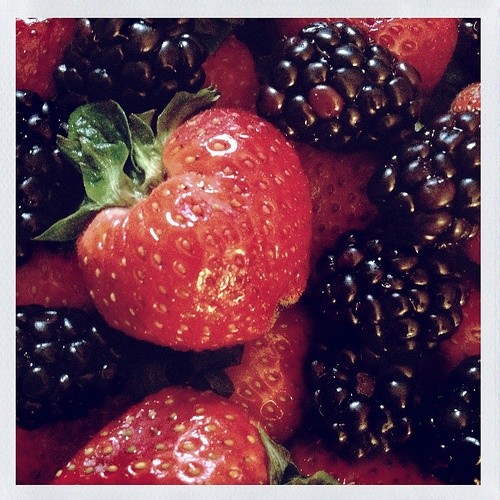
top-left (15, 18), bottom-right (481, 484)
